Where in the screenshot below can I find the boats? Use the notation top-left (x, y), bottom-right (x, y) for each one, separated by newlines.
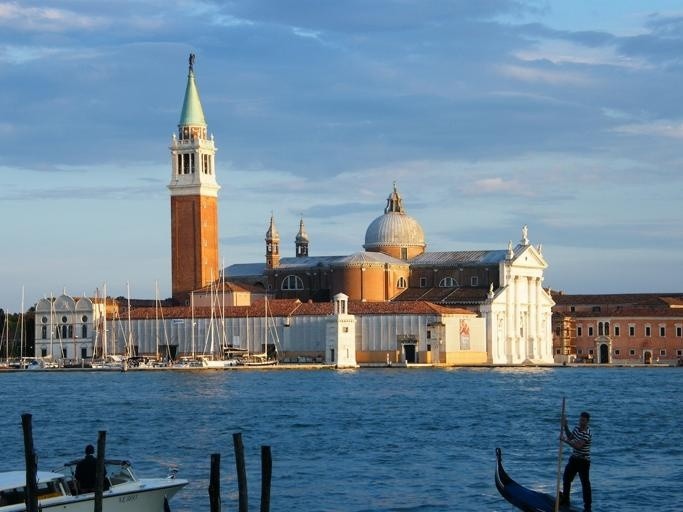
top-left (0, 456), bottom-right (192, 512)
top-left (494, 447), bottom-right (580, 512)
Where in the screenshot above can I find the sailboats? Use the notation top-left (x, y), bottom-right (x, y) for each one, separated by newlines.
top-left (0, 257), bottom-right (285, 371)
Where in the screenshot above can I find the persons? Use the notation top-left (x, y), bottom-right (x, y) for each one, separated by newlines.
top-left (555, 410), bottom-right (593, 512)
top-left (71, 443), bottom-right (109, 492)
